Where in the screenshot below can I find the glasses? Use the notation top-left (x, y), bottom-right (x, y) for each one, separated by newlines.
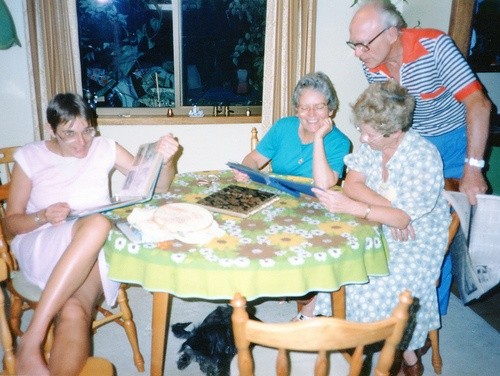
top-left (345, 26), bottom-right (389, 52)
top-left (295, 102), bottom-right (330, 117)
top-left (354, 122), bottom-right (386, 144)
top-left (56, 126), bottom-right (97, 142)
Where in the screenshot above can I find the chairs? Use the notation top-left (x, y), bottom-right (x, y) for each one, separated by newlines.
top-left (229, 289), bottom-right (413, 376)
top-left (299, 212), bottom-right (460, 373)
top-left (0, 146), bottom-right (145, 376)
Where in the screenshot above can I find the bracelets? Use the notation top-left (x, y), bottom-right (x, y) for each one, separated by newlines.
top-left (34, 212), bottom-right (43, 225)
top-left (465, 158), bottom-right (485, 168)
top-left (364, 205), bottom-right (371, 218)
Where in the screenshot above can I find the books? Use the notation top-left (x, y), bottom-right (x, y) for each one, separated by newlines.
top-left (196, 185), bottom-right (280, 218)
top-left (69, 143), bottom-right (163, 218)
top-left (227, 162), bottom-right (318, 197)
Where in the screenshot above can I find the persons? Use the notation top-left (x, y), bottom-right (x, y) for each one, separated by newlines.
top-left (311, 81), bottom-right (453, 376)
top-left (5, 94), bottom-right (180, 376)
top-left (234, 72), bottom-right (352, 322)
top-left (346, 0), bottom-right (492, 358)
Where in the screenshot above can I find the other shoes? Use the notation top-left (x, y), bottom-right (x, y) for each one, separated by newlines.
top-left (402, 351), bottom-right (424, 376)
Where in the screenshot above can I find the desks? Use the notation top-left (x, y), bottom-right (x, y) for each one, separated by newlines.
top-left (101, 171), bottom-right (390, 376)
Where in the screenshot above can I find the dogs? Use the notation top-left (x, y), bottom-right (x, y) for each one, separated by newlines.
top-left (170, 303), bottom-right (257, 376)
